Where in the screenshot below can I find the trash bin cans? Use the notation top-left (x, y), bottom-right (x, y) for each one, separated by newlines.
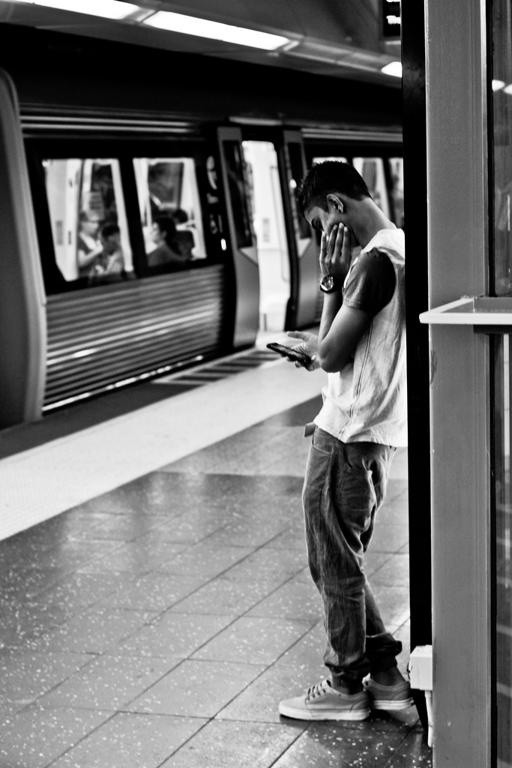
top-left (319, 275), bottom-right (340, 293)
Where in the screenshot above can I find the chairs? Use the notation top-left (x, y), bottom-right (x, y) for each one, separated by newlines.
top-left (363, 673), bottom-right (415, 711)
top-left (279, 679), bottom-right (370, 721)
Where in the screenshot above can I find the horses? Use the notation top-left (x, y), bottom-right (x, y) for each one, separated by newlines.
top-left (266, 342), bottom-right (311, 369)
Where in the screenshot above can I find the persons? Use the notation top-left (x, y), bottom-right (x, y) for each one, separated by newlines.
top-left (278, 160), bottom-right (412, 721)
top-left (77, 208), bottom-right (202, 281)
